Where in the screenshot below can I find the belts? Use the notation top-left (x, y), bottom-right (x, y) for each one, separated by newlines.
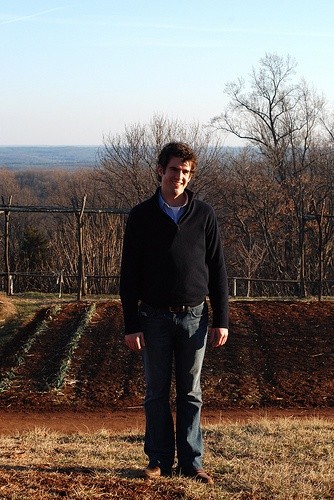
top-left (148, 301), bottom-right (204, 314)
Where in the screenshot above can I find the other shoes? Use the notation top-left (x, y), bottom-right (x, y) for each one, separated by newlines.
top-left (176, 465), bottom-right (212, 484)
top-left (140, 464), bottom-right (167, 477)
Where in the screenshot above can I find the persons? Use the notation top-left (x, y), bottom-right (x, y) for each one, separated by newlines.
top-left (116, 142), bottom-right (230, 483)
